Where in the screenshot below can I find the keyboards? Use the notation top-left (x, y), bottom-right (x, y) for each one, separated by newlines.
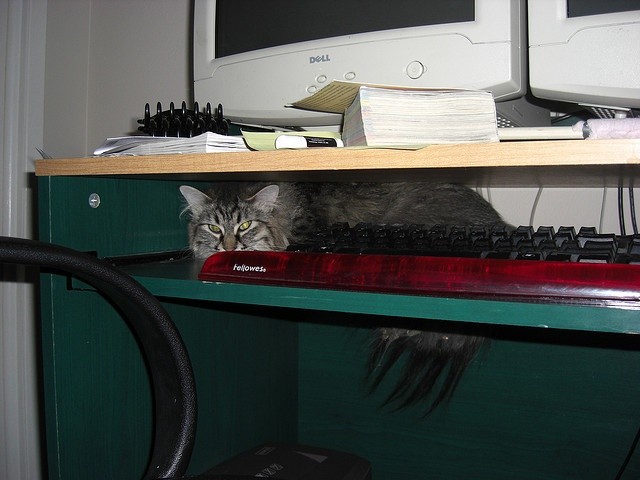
top-left (200, 220), bottom-right (640, 301)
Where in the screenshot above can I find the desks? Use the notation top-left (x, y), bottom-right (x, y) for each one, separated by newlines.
top-left (35, 137), bottom-right (640, 480)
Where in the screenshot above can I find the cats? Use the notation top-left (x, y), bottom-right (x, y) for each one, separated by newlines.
top-left (178, 179), bottom-right (517, 422)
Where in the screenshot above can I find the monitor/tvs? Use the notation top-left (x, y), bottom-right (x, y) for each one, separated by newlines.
top-left (193, 0), bottom-right (550, 133)
top-left (528, 0), bottom-right (640, 118)
